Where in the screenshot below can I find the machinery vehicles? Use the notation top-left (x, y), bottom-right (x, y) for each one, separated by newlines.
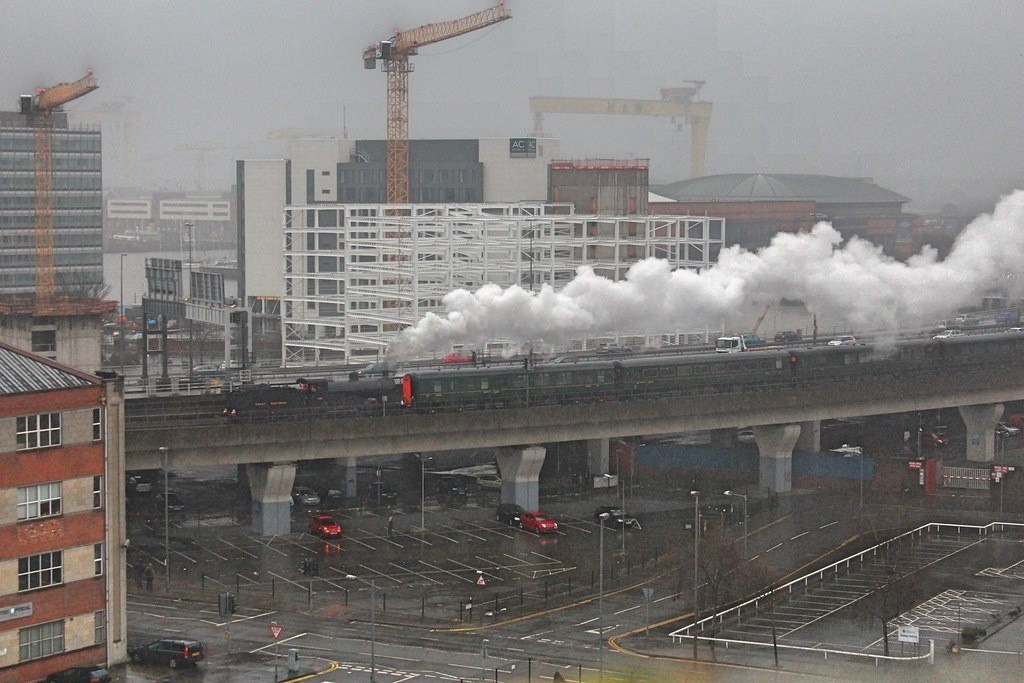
top-left (709, 304), bottom-right (767, 354)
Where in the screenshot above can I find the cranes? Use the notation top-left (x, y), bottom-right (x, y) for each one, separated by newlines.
top-left (358, 5), bottom-right (517, 332)
top-left (20, 68), bottom-right (100, 304)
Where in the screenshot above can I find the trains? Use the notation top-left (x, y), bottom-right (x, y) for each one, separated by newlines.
top-left (225, 325), bottom-right (1024, 424)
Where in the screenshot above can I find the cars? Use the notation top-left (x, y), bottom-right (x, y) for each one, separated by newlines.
top-left (220, 360), bottom-right (244, 369)
top-left (915, 431), bottom-right (952, 448)
top-left (291, 487), bottom-right (320, 506)
top-left (366, 482), bottom-right (398, 502)
top-left (189, 366), bottom-right (217, 375)
top-left (436, 476), bottom-right (471, 498)
top-left (552, 356), bottom-right (577, 363)
top-left (442, 351), bottom-right (473, 363)
top-left (595, 506), bottom-right (637, 529)
top-left (355, 364), bottom-right (397, 377)
top-left (496, 503), bottom-right (528, 527)
top-left (520, 512), bottom-right (558, 535)
top-left (308, 514), bottom-right (344, 537)
top-left (997, 413), bottom-right (1024, 435)
top-left (827, 334), bottom-right (857, 346)
top-left (477, 474), bottom-right (502, 489)
top-left (740, 328), bottom-right (803, 344)
top-left (927, 306), bottom-right (1024, 338)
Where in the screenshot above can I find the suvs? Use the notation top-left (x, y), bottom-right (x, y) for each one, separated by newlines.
top-left (47, 664), bottom-right (111, 683)
top-left (596, 342), bottom-right (632, 353)
top-left (129, 639), bottom-right (205, 670)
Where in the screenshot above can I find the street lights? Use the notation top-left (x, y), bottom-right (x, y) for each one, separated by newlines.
top-left (416, 453), bottom-right (432, 532)
top-left (690, 490), bottom-right (703, 662)
top-left (598, 512), bottom-right (609, 679)
top-left (159, 446), bottom-right (175, 591)
top-left (185, 222), bottom-right (195, 373)
top-left (723, 490), bottom-right (751, 565)
top-left (621, 440), bottom-right (645, 501)
top-left (120, 252), bottom-right (129, 371)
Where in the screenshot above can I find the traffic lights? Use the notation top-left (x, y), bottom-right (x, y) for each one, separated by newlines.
top-left (228, 595), bottom-right (238, 614)
